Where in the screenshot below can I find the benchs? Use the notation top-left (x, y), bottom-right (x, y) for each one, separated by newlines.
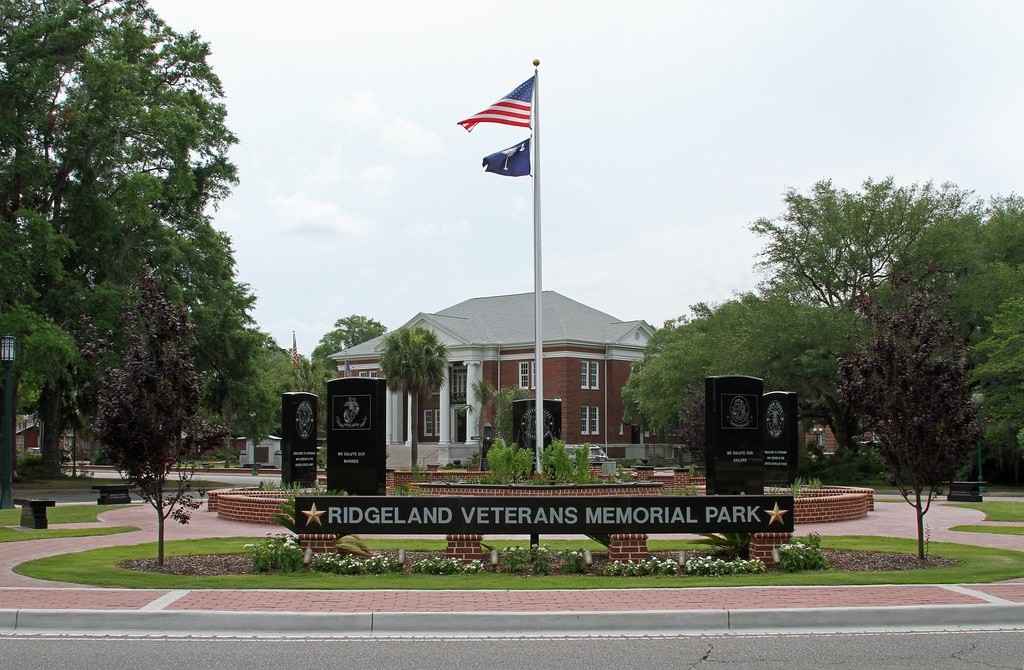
top-left (13, 498), bottom-right (57, 530)
top-left (92, 484), bottom-right (135, 505)
top-left (943, 478), bottom-right (989, 503)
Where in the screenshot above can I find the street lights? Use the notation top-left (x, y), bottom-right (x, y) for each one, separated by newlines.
top-left (70, 407), bottom-right (81, 477)
top-left (0, 334), bottom-right (17, 510)
top-left (970, 386), bottom-right (988, 493)
top-left (22, 414), bottom-right (29, 450)
top-left (248, 409), bottom-right (259, 476)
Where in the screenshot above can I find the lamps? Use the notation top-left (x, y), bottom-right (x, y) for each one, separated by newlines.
top-left (677, 550), bottom-right (687, 577)
top-left (771, 548), bottom-right (784, 571)
top-left (397, 548), bottom-right (407, 572)
top-left (302, 546), bottom-right (312, 566)
top-left (489, 548), bottom-right (500, 572)
top-left (583, 549), bottom-right (596, 576)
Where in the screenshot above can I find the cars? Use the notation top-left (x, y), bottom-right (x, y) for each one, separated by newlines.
top-left (859, 434), bottom-right (884, 452)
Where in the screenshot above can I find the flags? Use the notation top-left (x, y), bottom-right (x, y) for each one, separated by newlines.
top-left (344, 356), bottom-right (352, 377)
top-left (482, 138), bottom-right (531, 177)
top-left (457, 76), bottom-right (535, 133)
top-left (293, 337), bottom-right (297, 368)
